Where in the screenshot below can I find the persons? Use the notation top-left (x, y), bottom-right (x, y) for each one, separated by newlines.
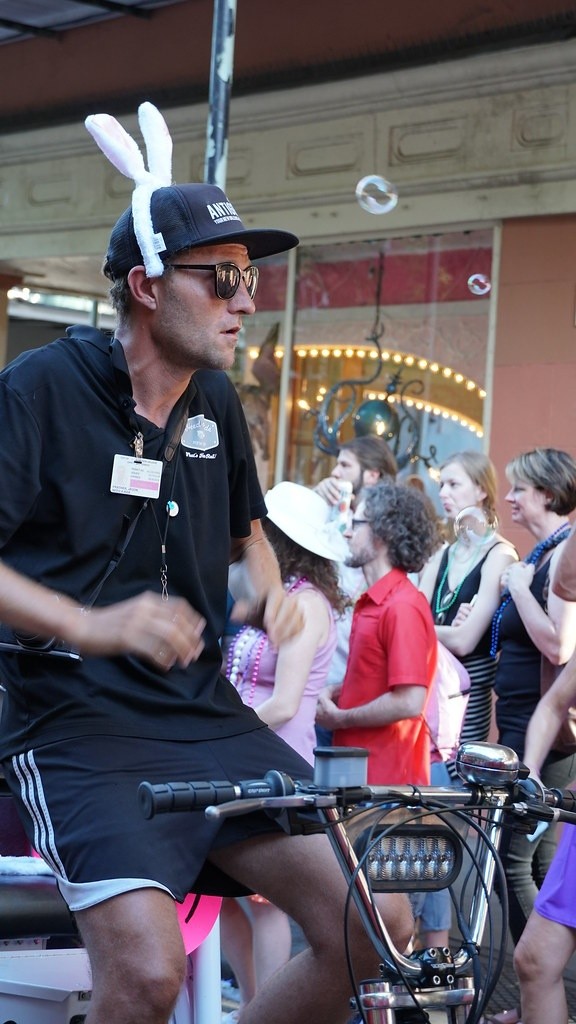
top-left (513, 531), bottom-right (576, 1024)
top-left (0, 185), bottom-right (415, 1024)
top-left (417, 452), bottom-right (521, 957)
top-left (220, 482), bottom-right (349, 1024)
top-left (314, 434), bottom-right (398, 506)
top-left (318, 482), bottom-right (437, 787)
top-left (497, 446), bottom-right (576, 946)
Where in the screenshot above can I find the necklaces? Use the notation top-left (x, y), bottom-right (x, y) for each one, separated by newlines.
top-left (435, 529), bottom-right (491, 612)
top-left (226, 577), bottom-right (307, 705)
top-left (491, 520), bottom-right (572, 658)
top-left (152, 439), bottom-right (187, 601)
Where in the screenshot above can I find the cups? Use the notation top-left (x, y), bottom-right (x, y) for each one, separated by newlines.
top-left (313, 746), bottom-right (369, 788)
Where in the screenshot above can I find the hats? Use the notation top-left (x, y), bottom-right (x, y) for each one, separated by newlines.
top-left (107, 183), bottom-right (300, 284)
top-left (263, 482), bottom-right (354, 563)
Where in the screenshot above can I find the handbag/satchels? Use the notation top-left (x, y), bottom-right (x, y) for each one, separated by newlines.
top-left (422, 641), bottom-right (471, 761)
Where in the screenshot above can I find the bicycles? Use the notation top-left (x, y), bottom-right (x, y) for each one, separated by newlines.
top-left (0, 740), bottom-right (575, 1023)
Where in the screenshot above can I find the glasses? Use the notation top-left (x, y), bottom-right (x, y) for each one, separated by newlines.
top-left (351, 519), bottom-right (370, 531)
top-left (163, 261), bottom-right (260, 301)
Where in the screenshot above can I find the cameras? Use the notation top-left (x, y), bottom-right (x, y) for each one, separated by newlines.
top-left (0, 619), bottom-right (84, 663)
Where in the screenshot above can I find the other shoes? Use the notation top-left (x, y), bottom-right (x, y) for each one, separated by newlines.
top-left (220, 978), bottom-right (241, 1002)
top-left (495, 1006), bottom-right (520, 1024)
top-left (223, 1010), bottom-right (239, 1024)
